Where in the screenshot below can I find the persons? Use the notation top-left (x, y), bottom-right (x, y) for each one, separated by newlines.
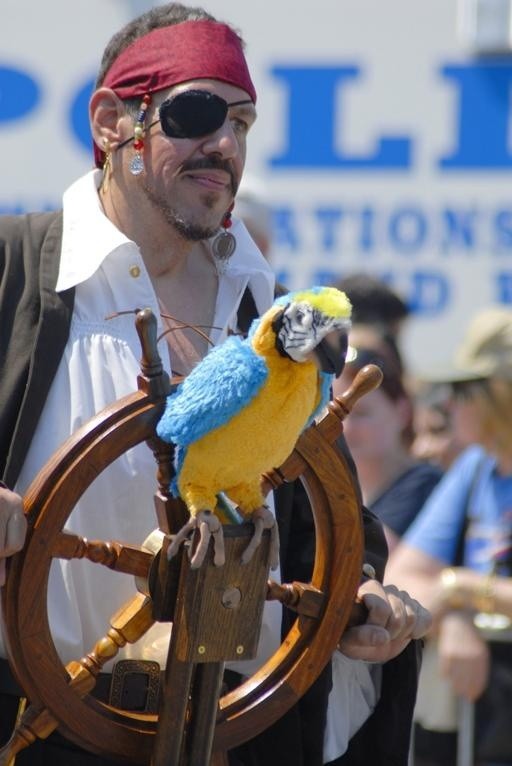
top-left (235, 203), bottom-right (512, 766)
top-left (0, 4), bottom-right (438, 765)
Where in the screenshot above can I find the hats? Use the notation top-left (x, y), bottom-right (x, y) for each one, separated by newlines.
top-left (424, 305), bottom-right (511, 382)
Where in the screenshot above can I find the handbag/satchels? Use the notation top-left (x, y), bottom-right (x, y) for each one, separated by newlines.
top-left (414, 622), bottom-right (462, 734)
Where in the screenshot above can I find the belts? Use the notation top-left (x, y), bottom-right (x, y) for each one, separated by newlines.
top-left (0, 655), bottom-right (166, 720)
